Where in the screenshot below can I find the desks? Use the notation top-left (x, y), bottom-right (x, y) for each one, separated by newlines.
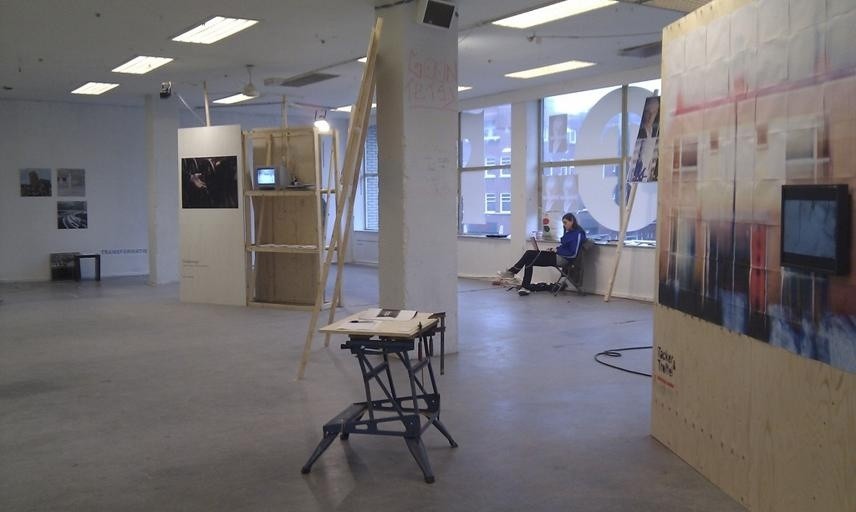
top-left (302, 308), bottom-right (458, 485)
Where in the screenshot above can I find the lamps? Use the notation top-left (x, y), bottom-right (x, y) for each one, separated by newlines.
top-left (311, 108), bottom-right (330, 133)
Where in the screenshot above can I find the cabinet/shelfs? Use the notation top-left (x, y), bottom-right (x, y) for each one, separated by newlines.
top-left (242, 126), bottom-right (324, 312)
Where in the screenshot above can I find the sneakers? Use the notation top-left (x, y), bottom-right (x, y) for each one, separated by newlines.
top-left (496, 271), bottom-right (513, 278)
top-left (518, 287), bottom-right (530, 294)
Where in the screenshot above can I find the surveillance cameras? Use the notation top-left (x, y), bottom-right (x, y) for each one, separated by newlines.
top-left (242, 85), bottom-right (258, 97)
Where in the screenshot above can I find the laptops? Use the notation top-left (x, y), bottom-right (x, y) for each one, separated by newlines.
top-left (530, 238), bottom-right (554, 254)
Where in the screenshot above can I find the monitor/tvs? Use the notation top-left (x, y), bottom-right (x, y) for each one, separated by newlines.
top-left (780, 183), bottom-right (852, 277)
top-left (256, 166), bottom-right (278, 191)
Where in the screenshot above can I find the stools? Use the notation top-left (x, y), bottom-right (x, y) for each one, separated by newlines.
top-left (73, 253), bottom-right (101, 281)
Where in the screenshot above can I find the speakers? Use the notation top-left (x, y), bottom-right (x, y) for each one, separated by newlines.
top-left (417, 0), bottom-right (457, 33)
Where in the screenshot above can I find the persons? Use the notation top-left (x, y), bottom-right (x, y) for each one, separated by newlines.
top-left (547, 117), bottom-right (567, 152)
top-left (495, 212), bottom-right (585, 297)
top-left (21, 171), bottom-right (49, 196)
top-left (638, 98), bottom-right (659, 138)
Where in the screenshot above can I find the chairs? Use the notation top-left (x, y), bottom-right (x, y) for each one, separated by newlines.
top-left (550, 240), bottom-right (590, 297)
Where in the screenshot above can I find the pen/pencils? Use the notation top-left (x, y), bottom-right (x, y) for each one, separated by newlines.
top-left (349, 321), bottom-right (374, 323)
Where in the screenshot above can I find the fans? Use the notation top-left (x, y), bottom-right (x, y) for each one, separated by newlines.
top-left (207, 64), bottom-right (302, 107)
